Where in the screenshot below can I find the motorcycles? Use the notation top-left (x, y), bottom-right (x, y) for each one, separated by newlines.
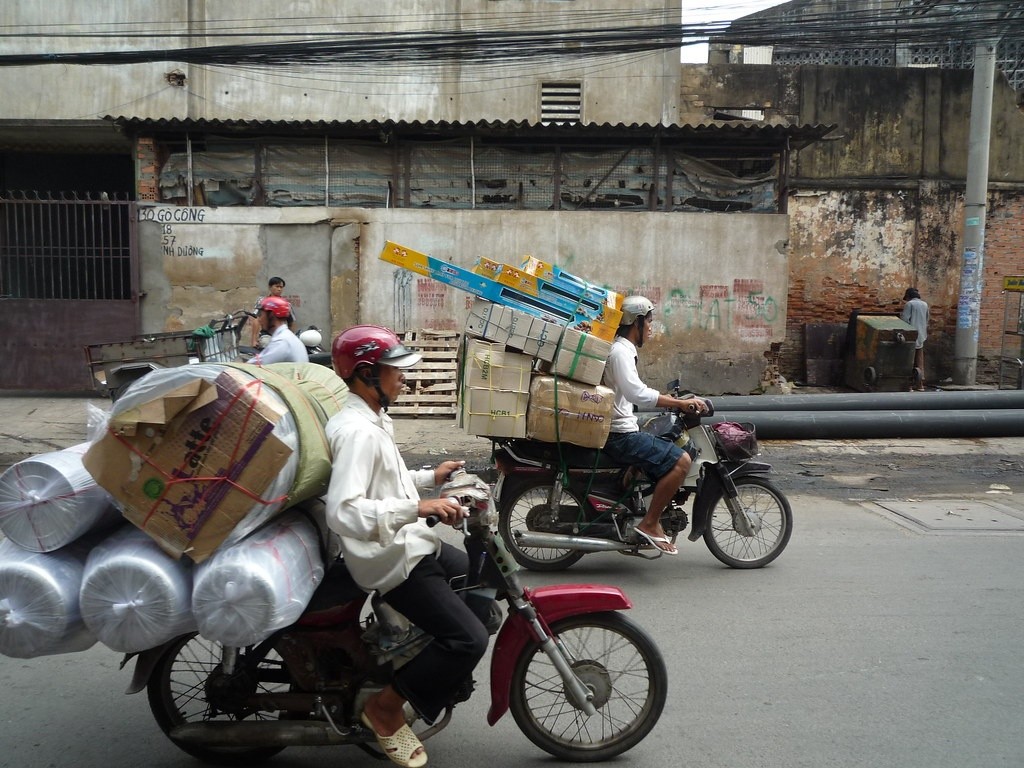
top-left (116, 464), bottom-right (668, 768)
top-left (233, 325), bottom-right (333, 369)
top-left (476, 378), bottom-right (794, 574)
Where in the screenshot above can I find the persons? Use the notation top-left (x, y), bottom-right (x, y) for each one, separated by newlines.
top-left (601, 296), bottom-right (709, 555)
top-left (323, 324), bottom-right (503, 768)
top-left (246, 297), bottom-right (308, 364)
top-left (900, 288), bottom-right (929, 392)
top-left (252, 276), bottom-right (296, 347)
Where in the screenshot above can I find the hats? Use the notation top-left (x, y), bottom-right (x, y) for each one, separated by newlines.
top-left (903, 287), bottom-right (918, 300)
top-left (377, 353), bottom-right (422, 367)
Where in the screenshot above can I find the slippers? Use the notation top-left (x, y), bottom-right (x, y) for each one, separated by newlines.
top-left (634, 526), bottom-right (679, 555)
top-left (917, 388), bottom-right (925, 392)
top-left (909, 389), bottom-right (913, 392)
top-left (362, 712), bottom-right (428, 767)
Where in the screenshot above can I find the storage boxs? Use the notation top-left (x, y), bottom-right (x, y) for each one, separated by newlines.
top-left (526, 376), bottom-right (615, 448)
top-left (492, 263), bottom-right (623, 329)
top-left (378, 241), bottom-right (616, 344)
top-left (463, 298), bottom-right (504, 341)
top-left (471, 255), bottom-right (499, 279)
top-left (549, 327), bottom-right (612, 386)
top-left (463, 387), bottom-right (530, 439)
top-left (522, 318), bottom-right (564, 362)
top-left (463, 338), bottom-right (533, 392)
top-left (494, 305), bottom-right (534, 350)
top-left (520, 254), bottom-right (625, 310)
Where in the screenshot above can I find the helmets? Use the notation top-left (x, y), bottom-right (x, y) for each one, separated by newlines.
top-left (619, 295), bottom-right (654, 325)
top-left (254, 297), bottom-right (290, 319)
top-left (331, 325), bottom-right (413, 379)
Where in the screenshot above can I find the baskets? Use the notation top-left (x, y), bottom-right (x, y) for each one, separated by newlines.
top-left (713, 422), bottom-right (759, 458)
